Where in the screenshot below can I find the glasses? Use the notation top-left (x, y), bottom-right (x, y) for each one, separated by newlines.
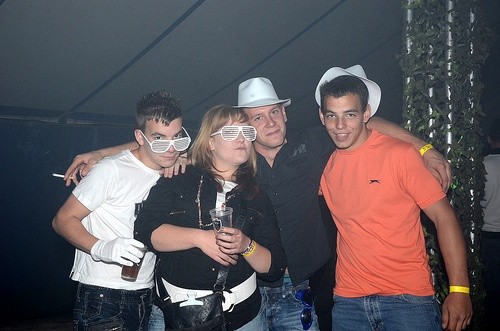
top-left (301, 288), bottom-right (314, 331)
top-left (210, 126), bottom-right (257, 141)
top-left (138, 127), bottom-right (191, 153)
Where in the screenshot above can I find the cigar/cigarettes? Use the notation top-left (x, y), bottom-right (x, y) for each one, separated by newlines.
top-left (52, 173), bottom-right (74, 181)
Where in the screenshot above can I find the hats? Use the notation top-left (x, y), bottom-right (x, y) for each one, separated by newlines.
top-left (314, 64), bottom-right (381, 117)
top-left (231, 77), bottom-right (291, 108)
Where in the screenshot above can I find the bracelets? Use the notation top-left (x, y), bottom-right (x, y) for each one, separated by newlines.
top-left (419, 144), bottom-right (434, 157)
top-left (240, 238), bottom-right (256, 255)
top-left (448, 285), bottom-right (470, 295)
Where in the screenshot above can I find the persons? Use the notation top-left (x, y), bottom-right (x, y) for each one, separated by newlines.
top-left (64, 77), bottom-right (452, 330)
top-left (133, 104), bottom-right (287, 330)
top-left (52, 89), bottom-right (191, 331)
top-left (479, 123), bottom-right (499, 309)
top-left (313, 64), bottom-right (473, 331)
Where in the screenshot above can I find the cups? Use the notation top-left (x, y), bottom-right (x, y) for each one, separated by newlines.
top-left (121, 245), bottom-right (148, 281)
top-left (209, 207), bottom-right (234, 236)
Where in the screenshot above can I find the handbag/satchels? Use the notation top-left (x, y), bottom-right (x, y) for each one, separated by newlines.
top-left (163, 292), bottom-right (225, 331)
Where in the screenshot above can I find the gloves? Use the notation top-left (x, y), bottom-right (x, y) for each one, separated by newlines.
top-left (90, 237), bottom-right (144, 266)
